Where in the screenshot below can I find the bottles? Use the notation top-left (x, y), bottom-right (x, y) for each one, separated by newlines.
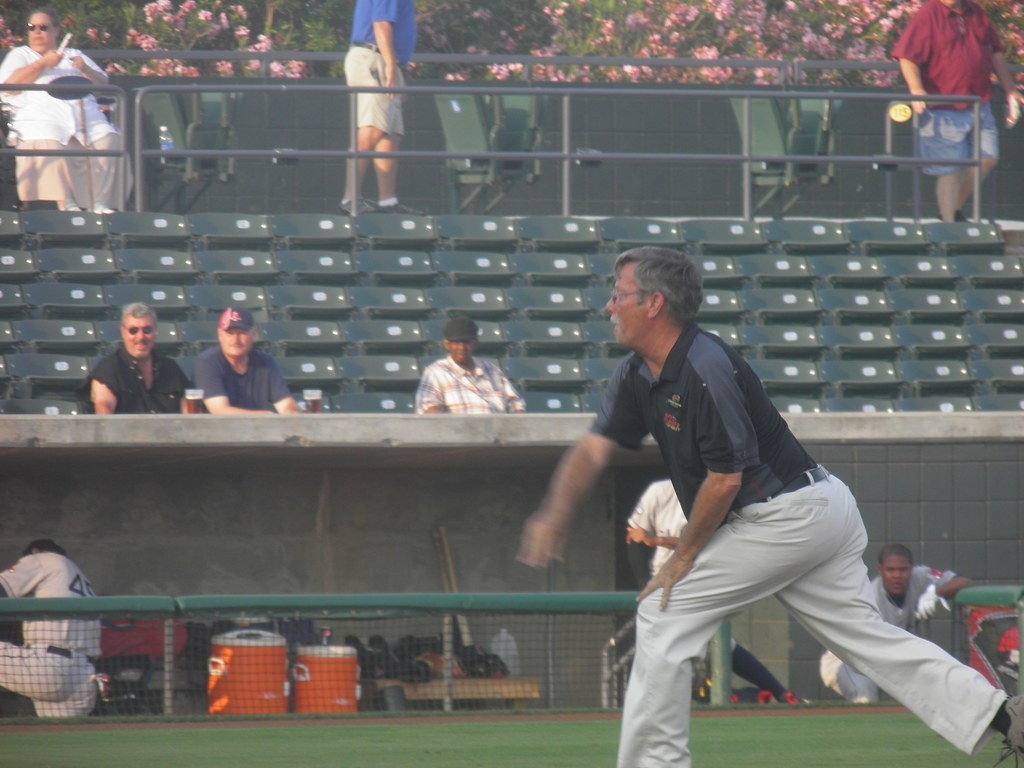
top-left (159, 125), bottom-right (174, 164)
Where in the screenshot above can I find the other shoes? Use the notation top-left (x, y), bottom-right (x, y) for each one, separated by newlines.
top-left (339, 198), bottom-right (382, 216)
top-left (58, 205), bottom-right (82, 212)
top-left (378, 204), bottom-right (424, 215)
top-left (85, 206), bottom-right (116, 214)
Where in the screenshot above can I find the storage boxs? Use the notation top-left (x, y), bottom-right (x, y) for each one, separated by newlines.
top-left (296, 643), bottom-right (364, 710)
top-left (210, 632), bottom-right (292, 716)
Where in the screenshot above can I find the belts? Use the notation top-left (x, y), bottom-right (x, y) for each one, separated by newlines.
top-left (730, 466), bottom-right (830, 510)
top-left (26, 643), bottom-right (94, 664)
top-left (352, 41), bottom-right (380, 55)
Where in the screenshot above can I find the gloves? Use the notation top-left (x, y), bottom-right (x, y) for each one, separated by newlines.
top-left (917, 585), bottom-right (938, 620)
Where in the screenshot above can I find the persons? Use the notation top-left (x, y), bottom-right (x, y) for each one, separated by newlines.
top-left (190, 306), bottom-right (302, 415)
top-left (891, 0), bottom-right (1024, 222)
top-left (415, 317), bottom-right (526, 414)
top-left (820, 543), bottom-right (975, 703)
top-left (626, 480), bottom-right (810, 704)
top-left (338, 0), bottom-right (424, 215)
top-left (1, 539), bottom-right (100, 718)
top-left (0, 8), bottom-right (134, 213)
top-left (516, 245), bottom-right (1024, 768)
top-left (82, 302), bottom-right (206, 414)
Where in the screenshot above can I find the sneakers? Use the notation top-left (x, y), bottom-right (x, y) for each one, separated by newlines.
top-left (1002, 696), bottom-right (1024, 768)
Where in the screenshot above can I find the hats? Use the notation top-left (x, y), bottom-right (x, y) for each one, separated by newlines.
top-left (22, 539), bottom-right (67, 555)
top-left (218, 308), bottom-right (254, 332)
top-left (445, 317), bottom-right (479, 340)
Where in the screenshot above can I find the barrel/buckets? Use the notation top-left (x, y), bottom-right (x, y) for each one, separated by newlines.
top-left (207, 630), bottom-right (289, 716)
top-left (293, 647), bottom-right (357, 713)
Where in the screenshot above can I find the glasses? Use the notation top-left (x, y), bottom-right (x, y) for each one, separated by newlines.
top-left (27, 23), bottom-right (48, 31)
top-left (121, 325), bottom-right (153, 334)
top-left (613, 290), bottom-right (645, 304)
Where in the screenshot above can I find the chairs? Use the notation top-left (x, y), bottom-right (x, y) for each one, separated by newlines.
top-left (0, 82), bottom-right (1024, 417)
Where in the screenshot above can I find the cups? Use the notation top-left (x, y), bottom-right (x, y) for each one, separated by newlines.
top-left (185, 389), bottom-right (204, 413)
top-left (303, 389), bottom-right (321, 414)
